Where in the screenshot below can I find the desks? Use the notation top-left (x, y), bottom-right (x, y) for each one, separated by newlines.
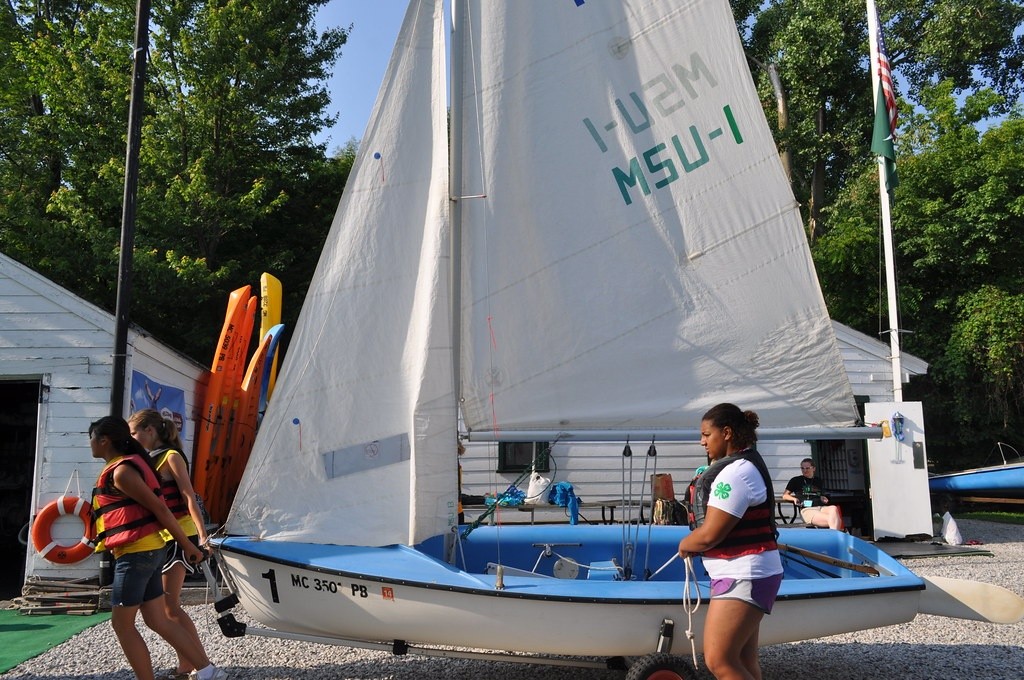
top-left (462, 499), bottom-right (654, 525)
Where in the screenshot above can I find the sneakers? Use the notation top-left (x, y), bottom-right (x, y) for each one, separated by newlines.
top-left (189, 668), bottom-right (229, 680)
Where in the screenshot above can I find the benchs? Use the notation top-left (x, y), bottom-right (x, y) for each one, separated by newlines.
top-left (772, 499), bottom-right (828, 529)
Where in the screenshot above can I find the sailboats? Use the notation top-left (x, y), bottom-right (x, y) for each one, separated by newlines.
top-left (219, 0), bottom-right (924, 655)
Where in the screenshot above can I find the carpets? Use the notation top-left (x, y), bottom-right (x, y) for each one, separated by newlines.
top-left (0, 609), bottom-right (114, 675)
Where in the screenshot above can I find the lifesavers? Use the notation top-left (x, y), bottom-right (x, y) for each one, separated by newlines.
top-left (32, 496), bottom-right (96, 564)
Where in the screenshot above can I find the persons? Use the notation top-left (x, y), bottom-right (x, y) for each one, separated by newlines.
top-left (679, 404), bottom-right (784, 680)
top-left (785, 459), bottom-right (843, 531)
top-left (89, 416), bottom-right (233, 680)
top-left (127, 409), bottom-right (215, 680)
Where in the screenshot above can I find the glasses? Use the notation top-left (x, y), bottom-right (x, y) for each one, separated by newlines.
top-left (800, 466), bottom-right (814, 470)
